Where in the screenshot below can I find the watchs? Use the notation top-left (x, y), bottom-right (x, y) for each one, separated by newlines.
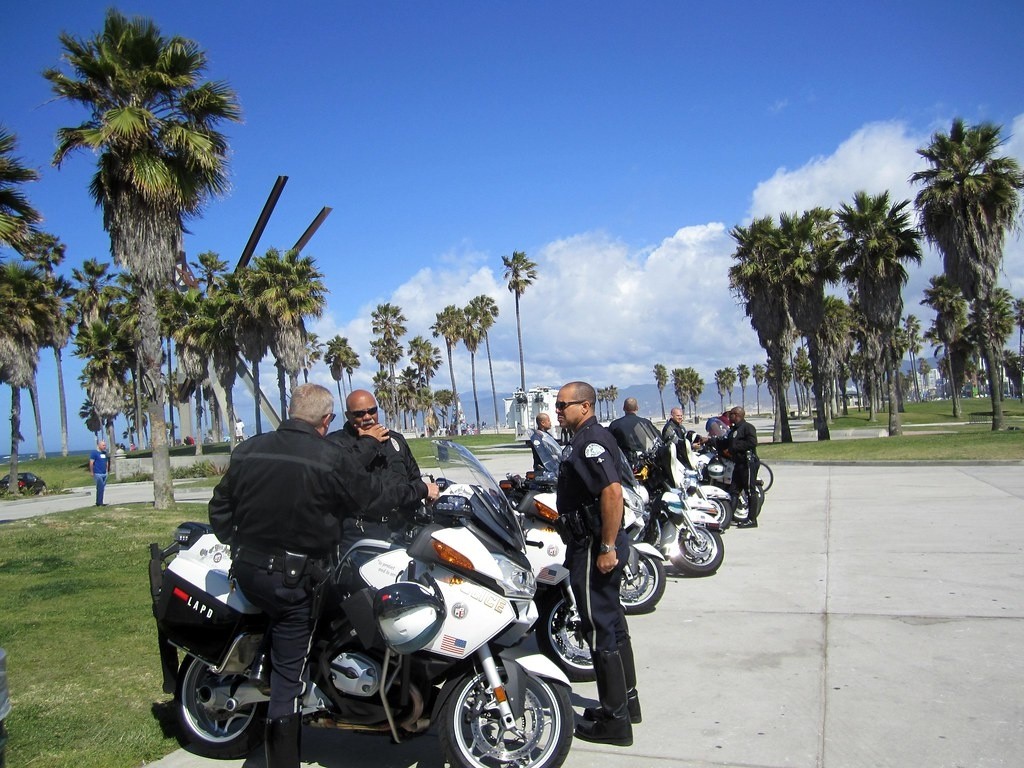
top-left (601, 544), bottom-right (617, 553)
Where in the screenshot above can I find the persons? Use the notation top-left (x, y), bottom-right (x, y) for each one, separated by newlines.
top-left (321, 392), bottom-right (422, 561)
top-left (208, 384), bottom-right (439, 768)
top-left (204, 433), bottom-right (213, 444)
top-left (130, 440), bottom-right (136, 451)
top-left (482, 421), bottom-right (486, 426)
top-left (608, 397), bottom-right (661, 468)
top-left (531, 413), bottom-right (558, 472)
top-left (184, 436), bottom-right (194, 446)
top-left (90, 441), bottom-right (109, 507)
top-left (236, 419), bottom-right (244, 442)
top-left (661, 407), bottom-right (700, 449)
top-left (517, 422), bottom-right (521, 436)
top-left (554, 381), bottom-right (642, 746)
top-left (698, 406), bottom-right (761, 528)
top-left (116, 443), bottom-right (125, 451)
top-left (429, 427), bottom-right (432, 431)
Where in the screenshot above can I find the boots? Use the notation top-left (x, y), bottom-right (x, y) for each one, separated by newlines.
top-left (738, 493), bottom-right (758, 528)
top-left (584, 639), bottom-right (641, 724)
top-left (576, 649), bottom-right (633, 745)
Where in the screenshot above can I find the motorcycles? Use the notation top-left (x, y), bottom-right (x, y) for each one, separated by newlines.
top-left (148, 439), bottom-right (575, 768)
top-left (421, 418), bottom-right (766, 685)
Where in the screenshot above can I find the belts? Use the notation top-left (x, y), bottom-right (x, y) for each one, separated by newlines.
top-left (235, 548), bottom-right (316, 573)
top-left (584, 517), bottom-right (603, 530)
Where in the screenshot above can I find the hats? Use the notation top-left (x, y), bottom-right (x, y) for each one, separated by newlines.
top-left (722, 404), bottom-right (735, 415)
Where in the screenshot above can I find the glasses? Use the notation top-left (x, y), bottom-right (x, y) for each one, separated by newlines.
top-left (322, 414), bottom-right (336, 421)
top-left (347, 405), bottom-right (378, 418)
top-left (555, 401), bottom-right (583, 410)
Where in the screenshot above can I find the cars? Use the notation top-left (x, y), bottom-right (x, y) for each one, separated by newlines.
top-left (0, 472), bottom-right (47, 495)
